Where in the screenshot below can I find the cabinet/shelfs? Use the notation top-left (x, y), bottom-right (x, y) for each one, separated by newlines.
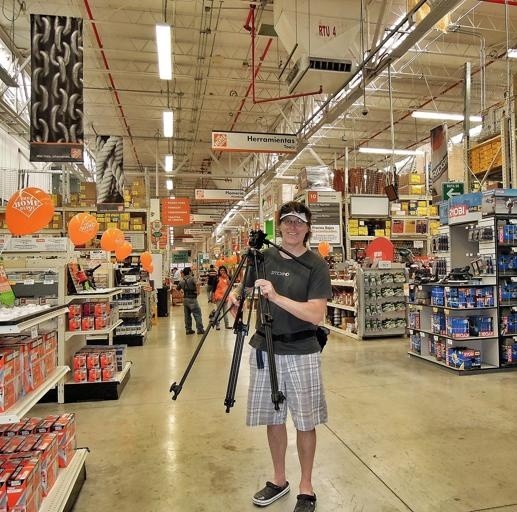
top-left (326, 269), bottom-right (409, 339)
top-left (308, 192), bottom-right (429, 262)
top-left (407, 218), bottom-right (517, 371)
top-left (0, 206), bottom-right (148, 251)
top-left (9, 265), bottom-right (132, 385)
top-left (94, 271), bottom-right (147, 337)
top-left (0, 302), bottom-right (91, 512)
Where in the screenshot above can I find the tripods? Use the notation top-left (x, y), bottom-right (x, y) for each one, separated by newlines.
top-left (169, 248), bottom-right (288, 413)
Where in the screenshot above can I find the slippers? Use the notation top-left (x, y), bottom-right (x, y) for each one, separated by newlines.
top-left (253, 481), bottom-right (290, 506)
top-left (294, 491), bottom-right (316, 512)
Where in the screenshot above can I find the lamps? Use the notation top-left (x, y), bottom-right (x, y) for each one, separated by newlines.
top-left (411, 111), bottom-right (482, 121)
top-left (359, 148), bottom-right (415, 155)
top-left (155, 0), bottom-right (175, 174)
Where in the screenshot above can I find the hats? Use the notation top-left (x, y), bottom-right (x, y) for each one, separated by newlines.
top-left (279, 209), bottom-right (308, 222)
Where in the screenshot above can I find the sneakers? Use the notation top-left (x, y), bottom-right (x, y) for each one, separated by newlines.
top-left (216, 324), bottom-right (220, 330)
top-left (186, 329), bottom-right (206, 334)
top-left (226, 325), bottom-right (233, 328)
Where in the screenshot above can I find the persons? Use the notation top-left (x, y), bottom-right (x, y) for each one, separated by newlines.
top-left (211, 265), bottom-right (233, 330)
top-left (205, 264), bottom-right (219, 304)
top-left (228, 201), bottom-right (333, 512)
top-left (170, 267), bottom-right (183, 286)
top-left (175, 267), bottom-right (206, 334)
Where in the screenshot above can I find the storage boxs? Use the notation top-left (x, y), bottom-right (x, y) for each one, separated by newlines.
top-left (471, 141), bottom-right (502, 173)
top-left (349, 173), bottom-right (439, 236)
top-left (3, 180), bottom-right (145, 231)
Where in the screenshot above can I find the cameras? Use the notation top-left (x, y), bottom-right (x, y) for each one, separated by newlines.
top-left (248, 228), bottom-right (265, 248)
